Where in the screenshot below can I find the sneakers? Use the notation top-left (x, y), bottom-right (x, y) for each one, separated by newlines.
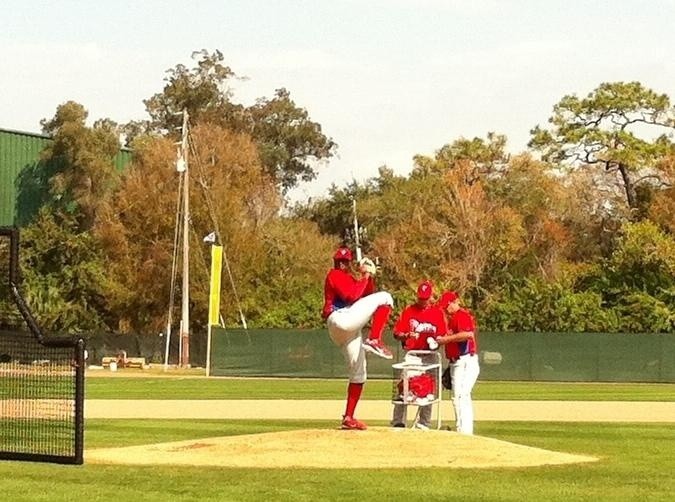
top-left (361, 337), bottom-right (394, 360)
top-left (342, 415), bottom-right (367, 429)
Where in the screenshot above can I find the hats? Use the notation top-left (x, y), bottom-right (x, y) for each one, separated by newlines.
top-left (438, 292), bottom-right (456, 309)
top-left (417, 282), bottom-right (431, 300)
top-left (334, 247), bottom-right (352, 263)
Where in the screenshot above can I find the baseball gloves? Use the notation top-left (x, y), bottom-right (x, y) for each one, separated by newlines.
top-left (356, 257), bottom-right (376, 274)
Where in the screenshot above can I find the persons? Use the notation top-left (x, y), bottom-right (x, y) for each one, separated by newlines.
top-left (392, 283), bottom-right (446, 427)
top-left (436, 293), bottom-right (480, 435)
top-left (322, 249), bottom-right (394, 430)
